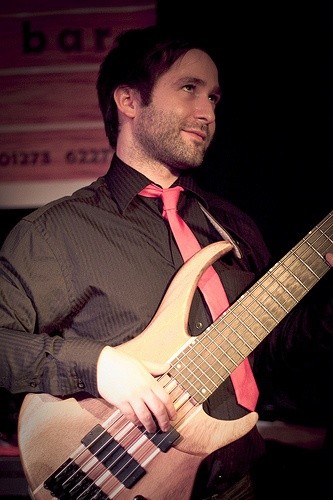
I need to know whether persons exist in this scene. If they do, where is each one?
[2,29,333,500]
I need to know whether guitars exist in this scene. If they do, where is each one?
[18,212,333,500]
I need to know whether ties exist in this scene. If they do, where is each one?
[140,185,259,412]
